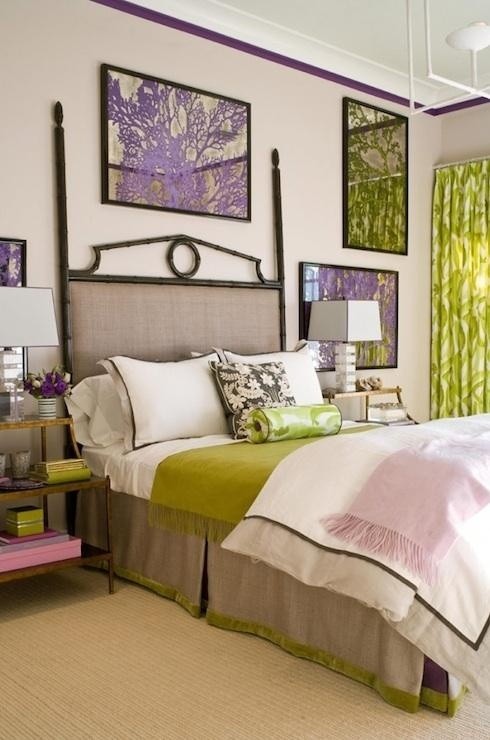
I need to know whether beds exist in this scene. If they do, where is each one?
[47,102,490,717]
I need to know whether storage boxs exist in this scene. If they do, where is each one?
[6,505,43,537]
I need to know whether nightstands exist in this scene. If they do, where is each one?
[322,387,419,426]
[1,415,113,593]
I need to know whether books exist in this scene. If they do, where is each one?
[29,458,91,484]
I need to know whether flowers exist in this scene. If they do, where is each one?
[22,364,72,399]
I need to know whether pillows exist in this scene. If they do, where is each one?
[96,351,233,454]
[210,340,323,405]
[247,404,341,444]
[210,360,297,440]
[64,375,124,448]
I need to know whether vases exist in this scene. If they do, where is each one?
[39,398,57,419]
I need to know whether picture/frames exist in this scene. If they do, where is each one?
[343,96,409,255]
[100,63,251,221]
[298,261,397,372]
[0,237,27,288]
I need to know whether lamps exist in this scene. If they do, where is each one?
[309,299,382,392]
[1,287,60,422]
[444,21,490,88]
